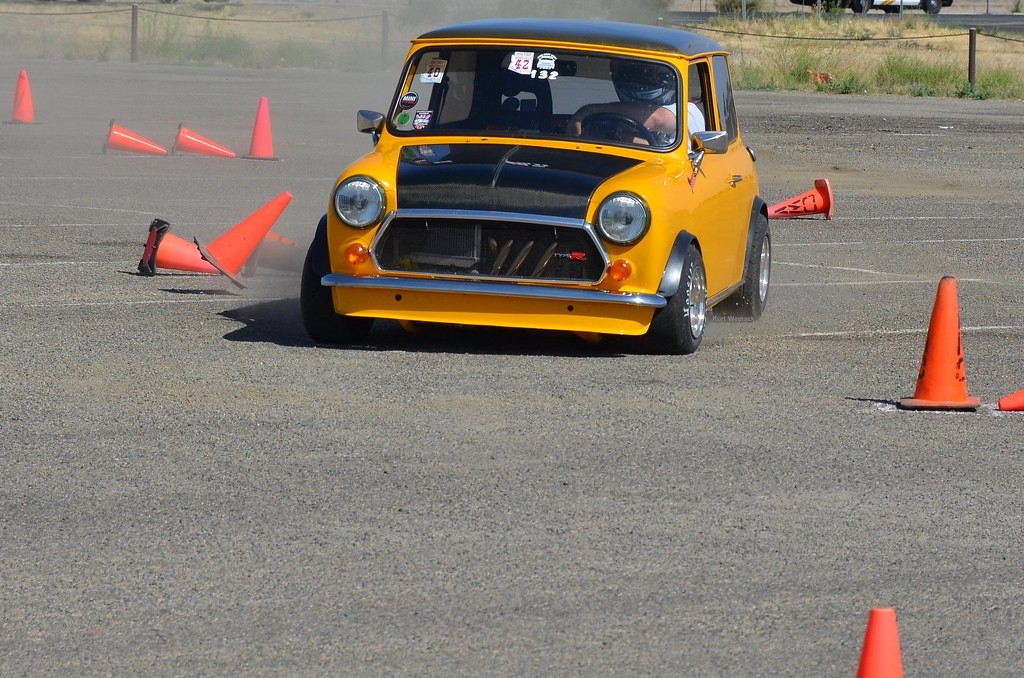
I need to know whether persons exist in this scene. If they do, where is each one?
[563,53,707,156]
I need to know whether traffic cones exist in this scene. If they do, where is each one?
[173,125,238,159]
[193,190,304,289]
[242,92,280,162]
[102,117,170,158]
[856,608,905,678]
[998,390,1024,412]
[137,217,223,279]
[7,68,43,127]
[767,178,835,221]
[895,275,984,412]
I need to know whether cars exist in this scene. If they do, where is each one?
[301,17,772,355]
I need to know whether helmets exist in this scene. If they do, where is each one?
[610,58,676,105]
